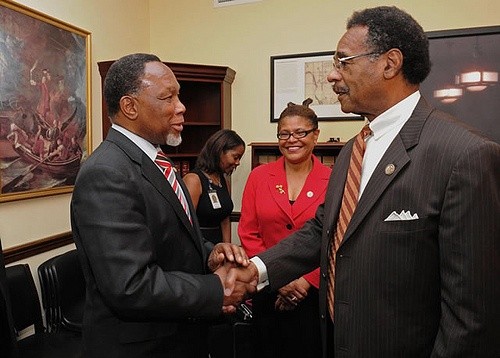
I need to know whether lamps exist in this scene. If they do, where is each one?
[431,37,498,107]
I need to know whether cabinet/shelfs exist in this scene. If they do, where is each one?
[97,60,237,180]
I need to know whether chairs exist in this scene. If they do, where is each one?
[6,250,88,358]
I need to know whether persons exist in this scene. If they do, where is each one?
[222,6,500,358]
[238,106,331,358]
[182,130,246,244]
[70,53,249,358]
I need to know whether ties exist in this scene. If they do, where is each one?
[156,152,193,228]
[326,124,374,325]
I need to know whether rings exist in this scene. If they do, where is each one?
[291,296,297,301]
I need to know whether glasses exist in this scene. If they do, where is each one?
[277,129,315,140]
[333,51,376,73]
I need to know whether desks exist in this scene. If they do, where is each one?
[247,138,347,178]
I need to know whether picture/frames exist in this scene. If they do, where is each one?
[419,24,500,148]
[0,0,93,201]
[270,49,364,123]
